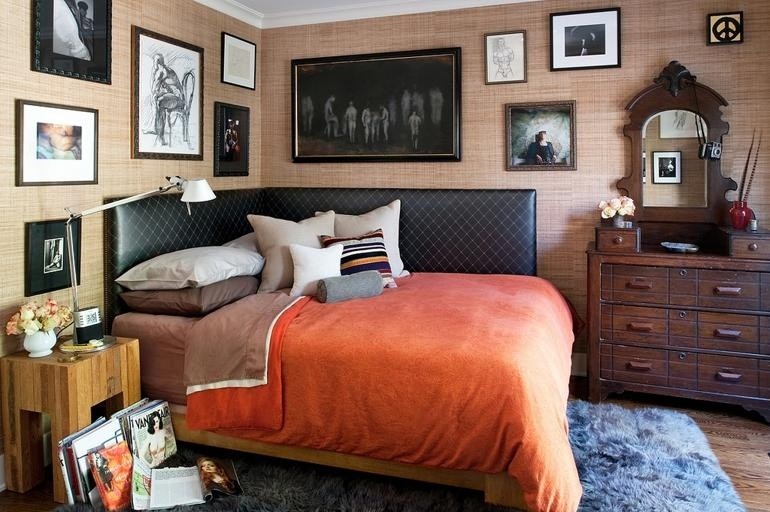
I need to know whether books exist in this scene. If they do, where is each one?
[57,397,245,512]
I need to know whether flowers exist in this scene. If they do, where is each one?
[7,301,71,333]
[598,196,635,218]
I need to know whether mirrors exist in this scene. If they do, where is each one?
[617,60,737,245]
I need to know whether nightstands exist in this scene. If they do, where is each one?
[0,335,141,504]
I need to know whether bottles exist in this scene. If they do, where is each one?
[751,219,757,231]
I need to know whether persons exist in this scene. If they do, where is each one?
[302,87,444,149]
[526,131,557,165]
[493,38,514,79]
[141,54,186,149]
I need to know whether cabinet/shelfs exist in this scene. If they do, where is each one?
[585,226,770,424]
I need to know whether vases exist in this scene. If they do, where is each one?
[614,212,626,228]
[24,332,57,358]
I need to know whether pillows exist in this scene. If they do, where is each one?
[115,199,410,313]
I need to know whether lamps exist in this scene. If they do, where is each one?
[60,175,217,351]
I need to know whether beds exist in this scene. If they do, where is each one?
[104,186,582,512]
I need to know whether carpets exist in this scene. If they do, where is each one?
[51,399,747,512]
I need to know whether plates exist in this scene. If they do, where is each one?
[658,240,701,254]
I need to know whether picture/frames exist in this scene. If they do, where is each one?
[15,96,101,186]
[707,11,744,44]
[27,1,116,89]
[210,100,253,178]
[292,46,462,163]
[659,111,707,140]
[505,100,578,170]
[24,217,81,296]
[550,8,621,70]
[128,21,207,165]
[653,151,682,184]
[219,29,256,91]
[484,29,527,84]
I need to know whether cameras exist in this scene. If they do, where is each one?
[698,143,707,159]
[706,141,722,160]
